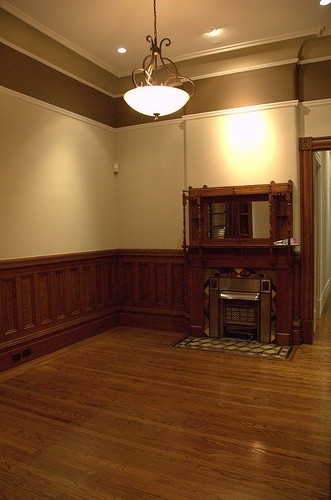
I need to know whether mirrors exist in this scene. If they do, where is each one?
[202,196,273,241]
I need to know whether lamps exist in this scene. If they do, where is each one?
[123,0,196,122]
